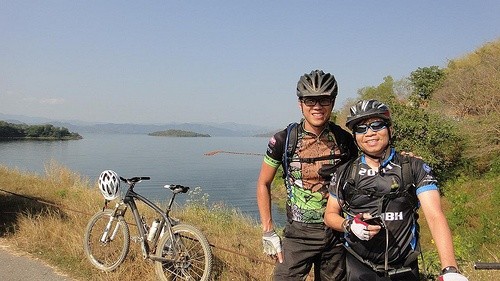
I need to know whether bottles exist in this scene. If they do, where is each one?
[147,218,160,243]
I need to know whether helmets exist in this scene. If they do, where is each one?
[98,170,121,200]
[297,70,338,97]
[346,99,391,130]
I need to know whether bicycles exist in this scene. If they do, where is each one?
[83,176,213,281]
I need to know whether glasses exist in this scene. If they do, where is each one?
[354,121,388,133]
[300,98,335,106]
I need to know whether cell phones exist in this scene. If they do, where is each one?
[363,217,385,231]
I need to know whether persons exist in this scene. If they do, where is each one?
[255,69,423,281]
[323,99,469,281]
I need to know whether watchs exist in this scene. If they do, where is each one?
[440,265,461,275]
